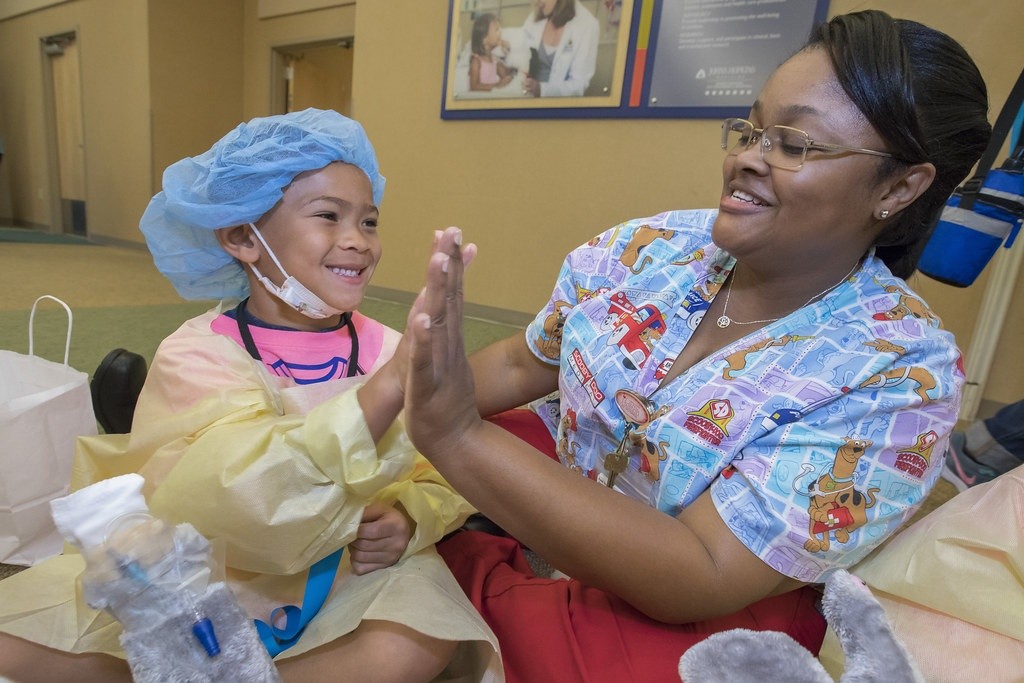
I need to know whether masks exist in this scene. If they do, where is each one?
[249,244,345,320]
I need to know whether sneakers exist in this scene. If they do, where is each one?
[940,431,998,493]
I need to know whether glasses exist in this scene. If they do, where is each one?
[720,118,937,168]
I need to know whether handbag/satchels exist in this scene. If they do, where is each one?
[0,295,99,569]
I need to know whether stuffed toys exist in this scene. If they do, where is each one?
[677,569,925,682]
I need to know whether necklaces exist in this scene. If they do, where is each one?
[716,258,859,328]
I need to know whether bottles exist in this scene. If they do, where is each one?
[977,146,1024,212]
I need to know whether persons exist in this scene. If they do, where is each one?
[467,13,512,91]
[0,9,994,683]
[507,0,601,97]
